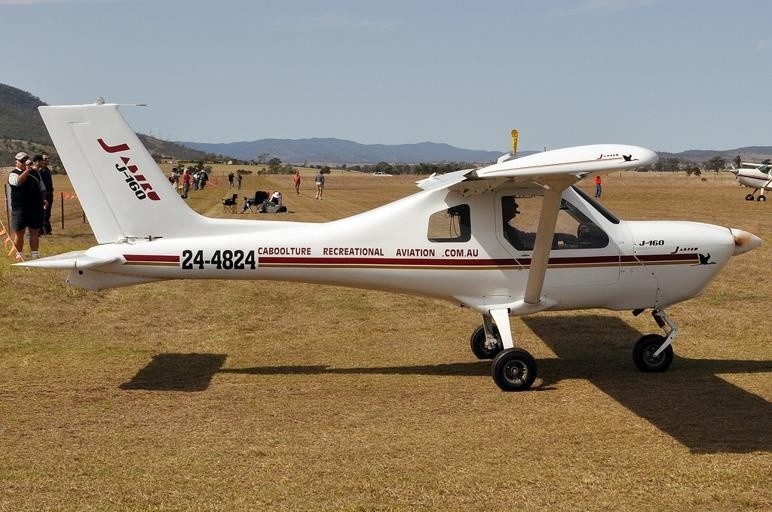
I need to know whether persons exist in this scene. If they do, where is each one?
[314,171,325,201]
[194,169,198,190]
[182,168,193,194]
[293,172,300,194]
[228,171,233,189]
[232,173,236,185]
[171,167,180,192]
[198,177,204,189]
[38,151,54,235]
[594,175,601,198]
[24,159,33,171]
[8,151,48,261]
[24,155,46,236]
[201,170,208,188]
[236,171,242,189]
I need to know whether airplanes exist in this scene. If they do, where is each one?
[12,103,763,392]
[729,163,772,202]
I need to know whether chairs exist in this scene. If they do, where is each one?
[222,193,238,214]
[243,190,266,214]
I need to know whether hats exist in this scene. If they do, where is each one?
[273,192,279,198]
[15,152,43,165]
[172,168,177,172]
